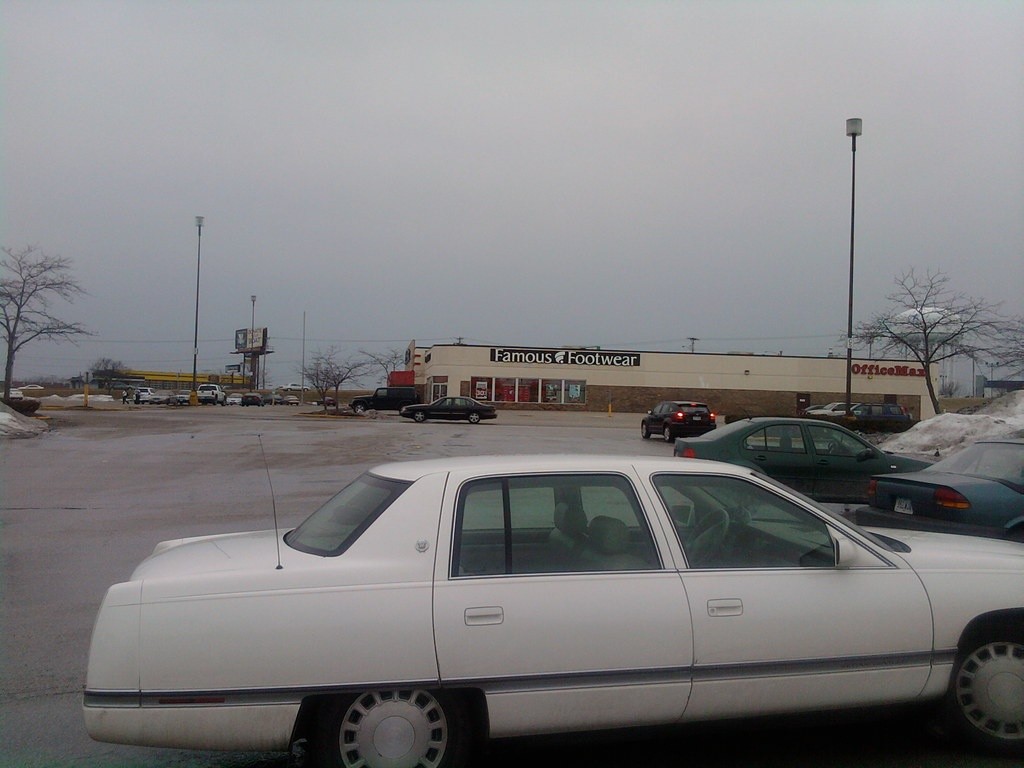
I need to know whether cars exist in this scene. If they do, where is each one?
[673,407,935,504]
[837,403,913,424]
[0,383,338,407]
[854,437,1023,533]
[805,401,862,416]
[640,400,717,442]
[399,396,497,425]
[81,434,1023,768]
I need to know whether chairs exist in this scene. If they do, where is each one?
[542,503,591,574]
[779,435,792,452]
[573,515,639,571]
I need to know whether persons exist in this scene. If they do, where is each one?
[135,388,141,401]
[122,389,129,404]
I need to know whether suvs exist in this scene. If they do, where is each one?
[347,386,418,414]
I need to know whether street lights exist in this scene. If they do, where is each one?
[250,294,257,392]
[188,214,203,406]
[845,116,863,415]
